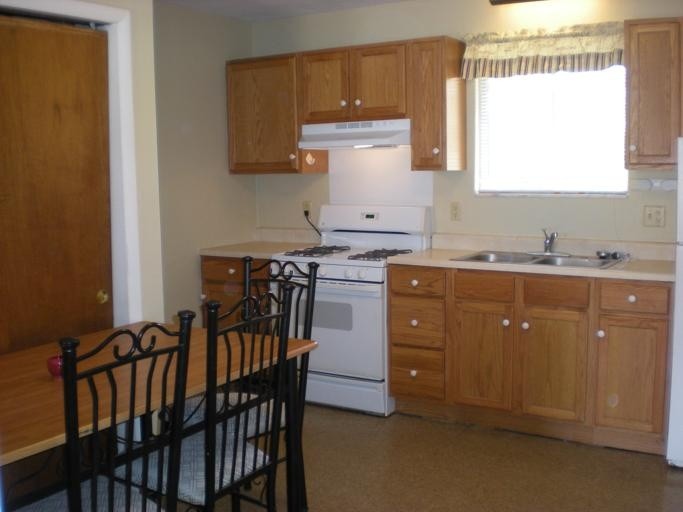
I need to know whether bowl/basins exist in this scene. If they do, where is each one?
[47,355,63,378]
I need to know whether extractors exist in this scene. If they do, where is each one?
[298,118,411,149]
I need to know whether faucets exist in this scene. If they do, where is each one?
[542,228,558,252]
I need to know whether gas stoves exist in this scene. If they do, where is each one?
[270,244,413,282]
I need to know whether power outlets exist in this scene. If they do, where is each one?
[300,198,314,220]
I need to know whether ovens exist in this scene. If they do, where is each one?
[270,276,396,417]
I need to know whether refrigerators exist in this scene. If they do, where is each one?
[664,136,682,470]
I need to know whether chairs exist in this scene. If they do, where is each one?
[182,255,321,512]
[101,280,297,512]
[2,304,196,512]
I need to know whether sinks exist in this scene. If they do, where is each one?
[532,255,622,270]
[449,250,538,265]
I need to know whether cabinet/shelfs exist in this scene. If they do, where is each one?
[224,53,329,175]
[448,268,591,428]
[410,35,467,173]
[384,261,446,405]
[199,255,270,336]
[624,14,679,167]
[592,275,671,435]
[301,40,409,124]
